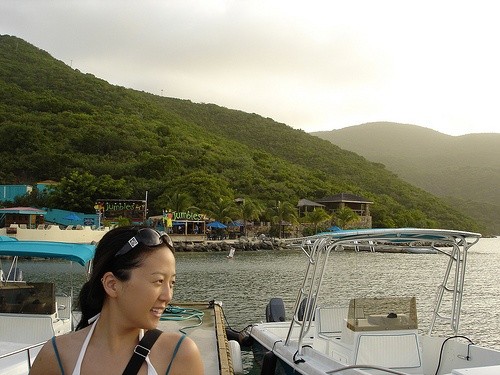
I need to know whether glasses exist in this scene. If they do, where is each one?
[115,229,173,258]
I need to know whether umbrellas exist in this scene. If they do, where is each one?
[206,221,228,241]
[225,218,253,238]
[325,225,342,232]
[274,219,293,240]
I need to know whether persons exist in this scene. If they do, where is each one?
[163,220,230,240]
[28,224,206,375]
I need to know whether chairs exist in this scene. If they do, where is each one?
[329,296,423,369]
[24,294,72,335]
[0,280,65,345]
[5,223,120,234]
[313,306,365,356]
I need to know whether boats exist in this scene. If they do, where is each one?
[1,235,242,375]
[245,225,499,375]
